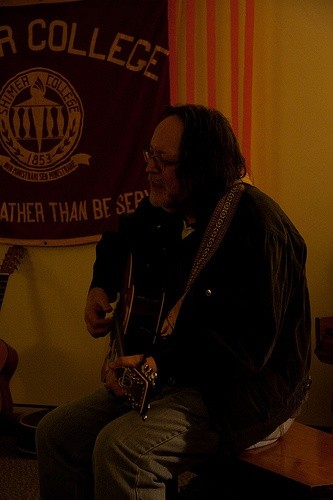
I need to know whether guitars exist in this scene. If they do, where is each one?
[1,243,28,443]
[101,258,156,417]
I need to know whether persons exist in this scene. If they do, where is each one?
[34,103,312,500]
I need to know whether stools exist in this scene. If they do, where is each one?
[165,420,333,499]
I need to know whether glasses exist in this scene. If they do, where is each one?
[144,145,178,172]
